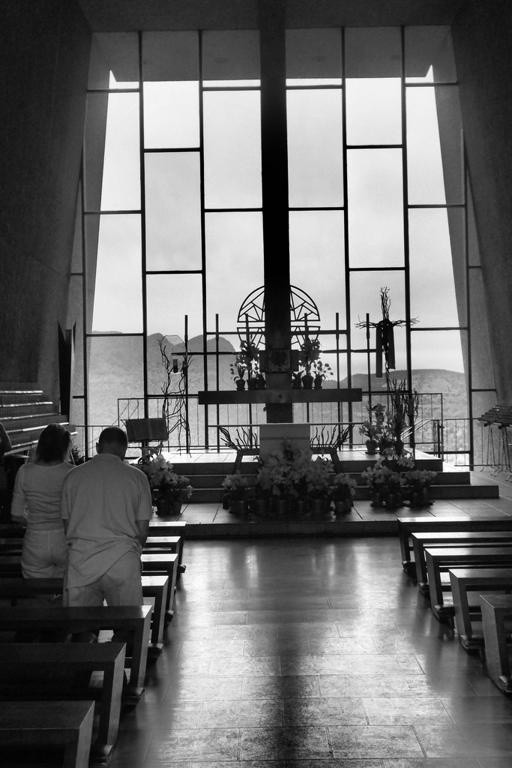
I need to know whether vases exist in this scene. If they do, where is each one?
[334,496,354,517]
[310,500,330,521]
[297,500,306,512]
[384,490,405,510]
[292,378,302,390]
[302,373,313,389]
[155,497,183,516]
[247,377,255,390]
[235,378,245,391]
[255,374,266,388]
[377,435,395,454]
[313,375,323,389]
[367,490,383,507]
[276,498,288,514]
[408,486,432,507]
[236,500,248,522]
[395,434,404,455]
[365,437,379,455]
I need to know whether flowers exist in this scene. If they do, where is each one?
[332,473,358,497]
[407,468,439,487]
[142,456,192,502]
[360,456,384,494]
[385,362,421,437]
[229,363,248,379]
[236,340,261,377]
[300,338,327,373]
[293,451,309,500]
[262,454,293,499]
[385,460,406,490]
[304,450,328,499]
[312,360,334,376]
[223,474,254,501]
[380,409,398,440]
[288,361,311,379]
[358,421,379,440]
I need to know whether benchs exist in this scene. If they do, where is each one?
[411,531,512,608]
[0,597,156,713]
[0,641,127,768]
[0,535,181,593]
[397,516,512,576]
[0,553,179,621]
[448,568,512,654]
[0,700,95,768]
[0,520,187,575]
[479,594,512,696]
[424,547,512,624]
[0,576,169,661]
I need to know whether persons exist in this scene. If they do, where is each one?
[11,423,77,579]
[60,426,151,634]
